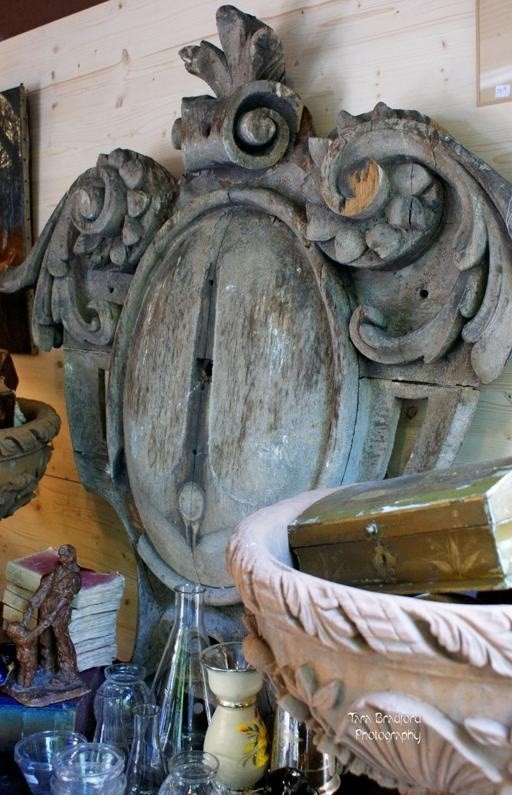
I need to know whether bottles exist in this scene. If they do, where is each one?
[94,583,272,795]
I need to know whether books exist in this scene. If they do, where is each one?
[2,546,126,673]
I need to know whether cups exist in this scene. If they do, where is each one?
[16,729,125,793]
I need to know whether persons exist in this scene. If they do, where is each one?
[6,605,49,689]
[29,544,80,687]
